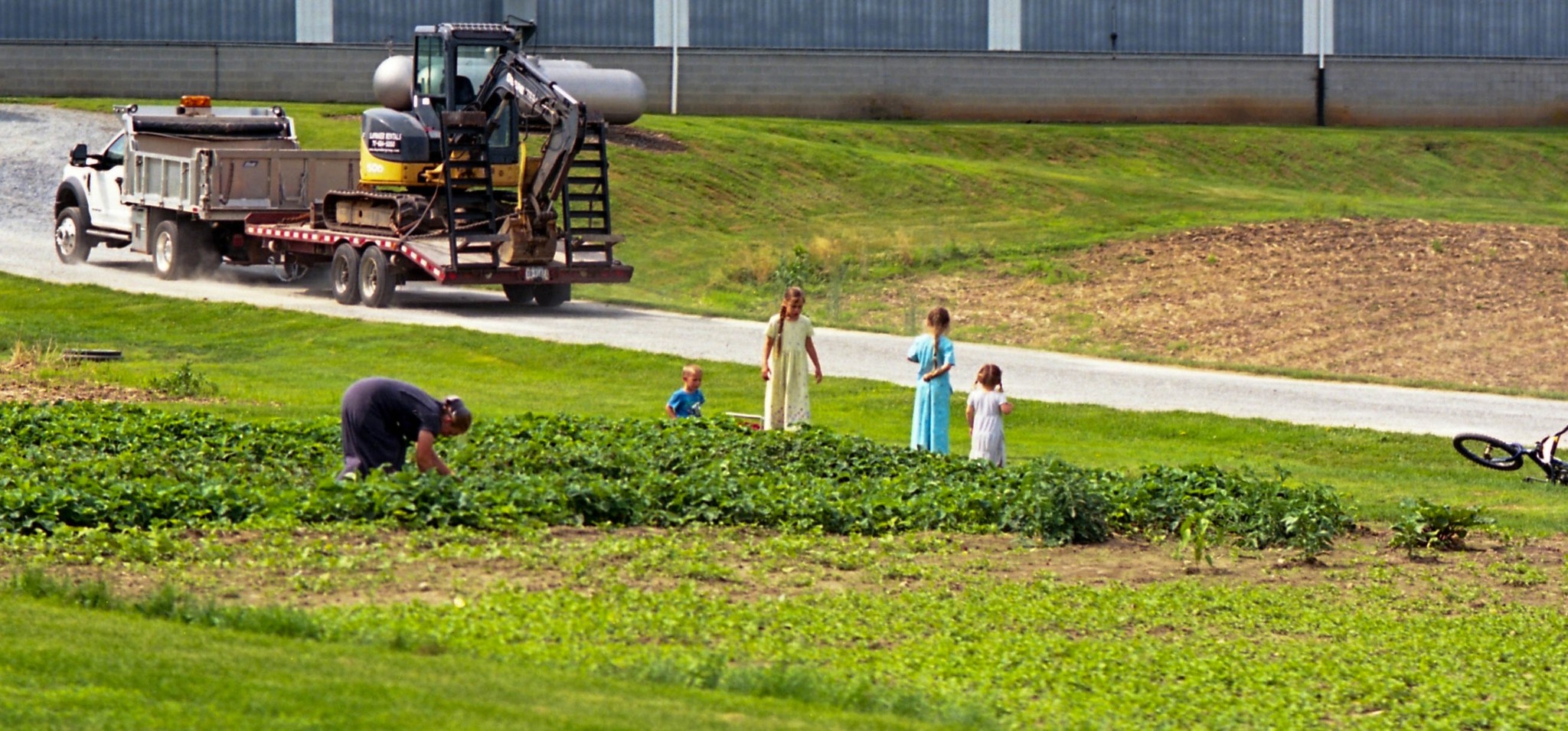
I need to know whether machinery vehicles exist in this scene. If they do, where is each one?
[323,13,588,265]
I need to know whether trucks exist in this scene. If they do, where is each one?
[54,95,373,281]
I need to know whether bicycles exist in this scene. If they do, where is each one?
[1453,425,1568,487]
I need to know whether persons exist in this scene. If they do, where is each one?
[762,287,823,431]
[907,307,956,455]
[334,376,472,485]
[967,364,1014,469]
[665,364,706,419]
